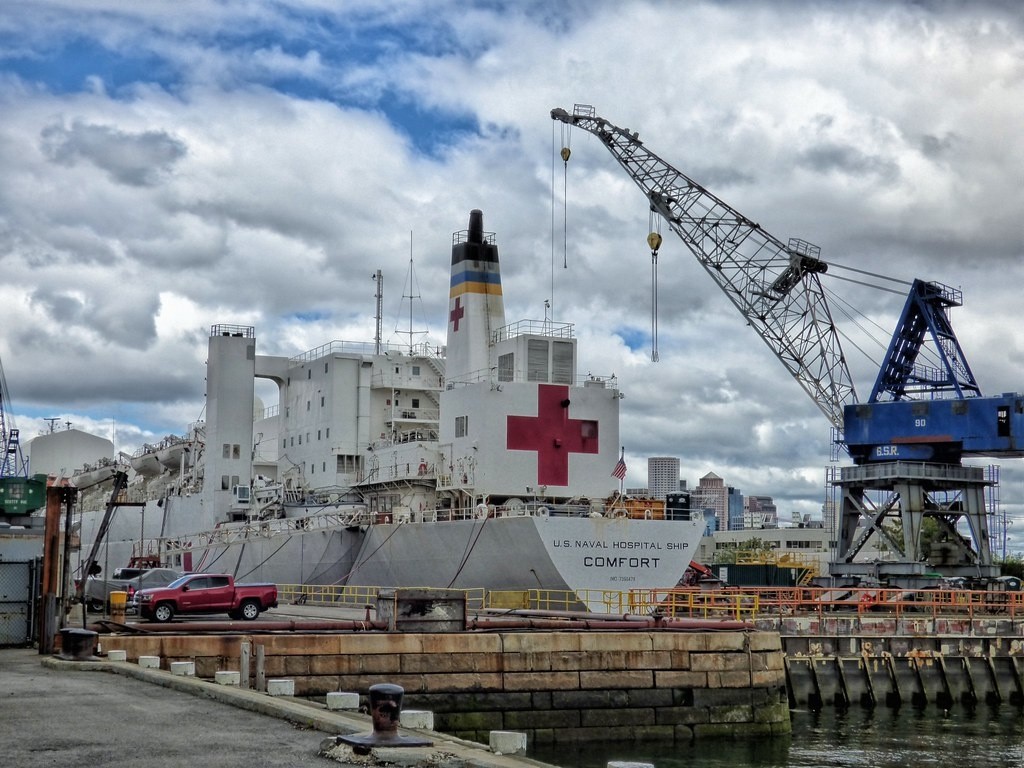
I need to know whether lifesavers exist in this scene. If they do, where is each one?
[613,507,629,518]
[418,463,428,475]
[476,503,489,519]
[861,594,873,608]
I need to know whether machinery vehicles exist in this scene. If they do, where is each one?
[551,107,1024,463]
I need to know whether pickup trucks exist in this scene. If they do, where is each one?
[134,573,280,623]
[76,563,185,614]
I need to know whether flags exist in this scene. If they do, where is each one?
[610,457,628,478]
